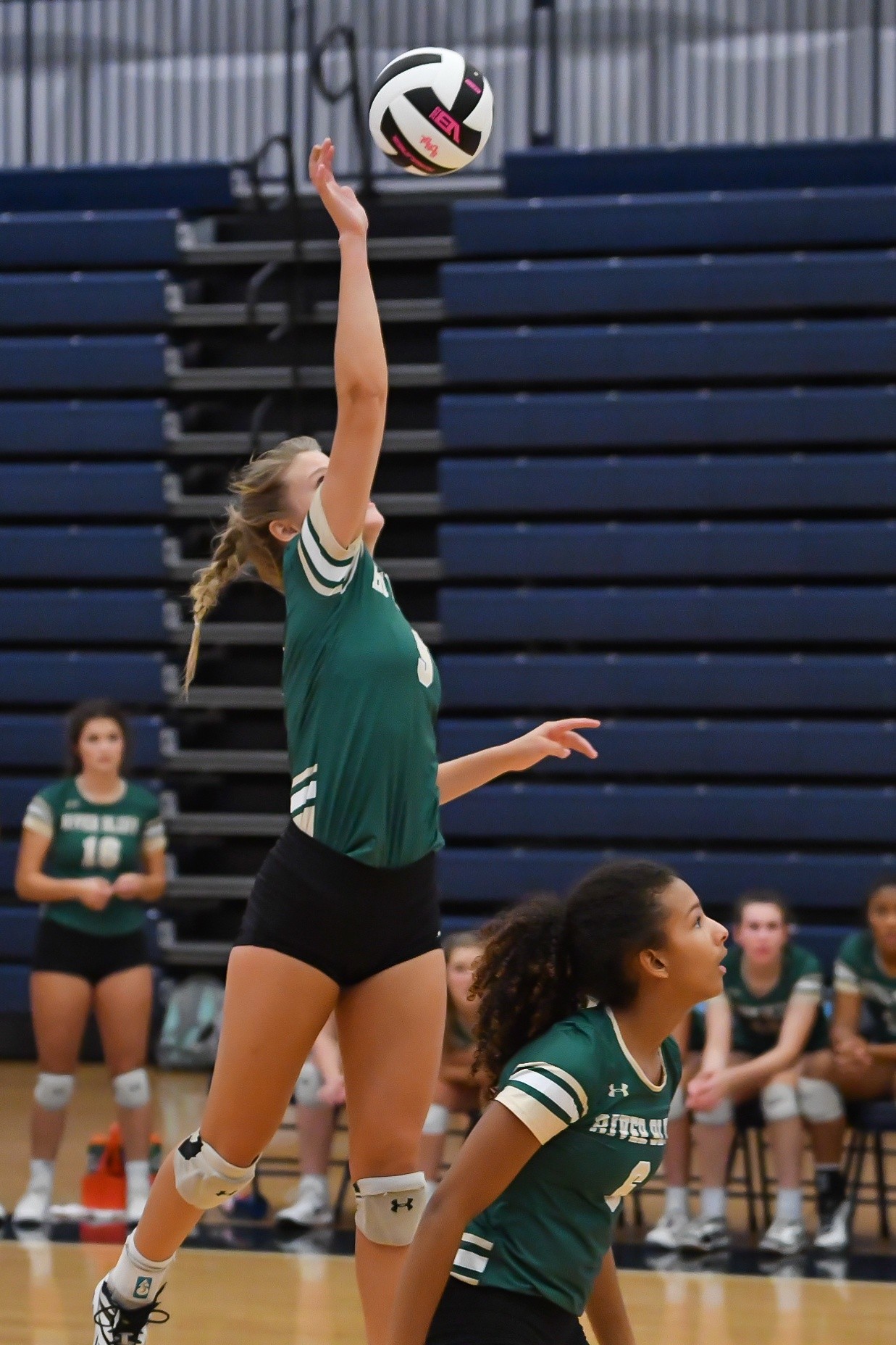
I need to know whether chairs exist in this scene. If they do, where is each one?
[187,989,896,1240]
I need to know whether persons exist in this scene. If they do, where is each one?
[390,858,729,1345]
[11,706,168,1229]
[93,136,603,1345]
[271,887,491,1231]
[675,890,834,1257]
[794,880,896,1254]
[644,1003,710,1250]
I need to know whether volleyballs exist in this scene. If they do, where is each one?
[368,46,495,178]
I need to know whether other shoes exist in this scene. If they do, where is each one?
[811,1169,852,1250]
[13,1231,49,1249]
[648,1252,682,1274]
[682,1253,727,1270]
[276,1229,332,1252]
[816,1257,848,1278]
[15,1195,46,1230]
[646,1214,687,1248]
[755,1219,806,1253]
[92,1274,169,1345]
[276,1193,331,1227]
[678,1215,729,1251]
[125,1199,147,1225]
[760,1256,804,1282]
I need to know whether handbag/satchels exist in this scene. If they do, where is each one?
[81,1123,158,1244]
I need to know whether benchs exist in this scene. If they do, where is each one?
[0,129,896,1048]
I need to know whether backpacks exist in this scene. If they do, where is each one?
[155,977,224,1068]
